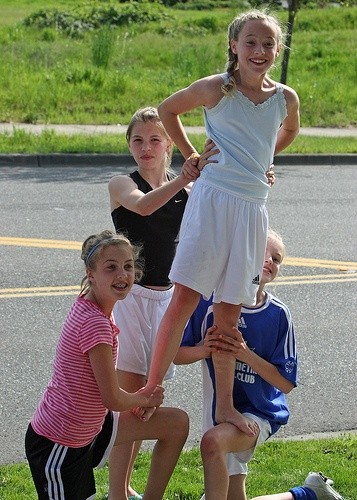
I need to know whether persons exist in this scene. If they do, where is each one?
[173,231,343,500]
[132,10,299,437]
[108,107,275,500]
[24,231,191,500]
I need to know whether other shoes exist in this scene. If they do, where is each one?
[303,470,343,500]
